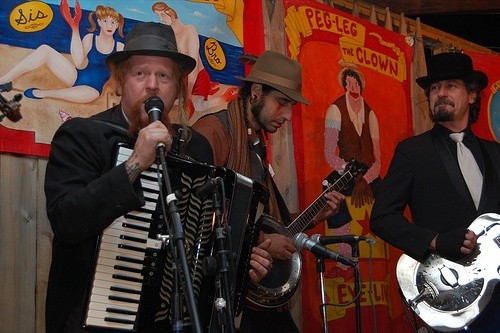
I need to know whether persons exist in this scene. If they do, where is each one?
[192,50,345,333]
[44,22,273,333]
[370,53,500,333]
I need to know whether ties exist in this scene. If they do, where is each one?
[450,132,483,211]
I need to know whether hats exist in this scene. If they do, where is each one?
[106,21,196,77]
[235,49,312,108]
[415,51,489,91]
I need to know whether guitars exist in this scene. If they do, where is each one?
[242,159,366,311]
[395,213,500,333]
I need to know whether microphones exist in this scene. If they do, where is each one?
[144,96,166,152]
[292,232,357,268]
[310,233,369,246]
